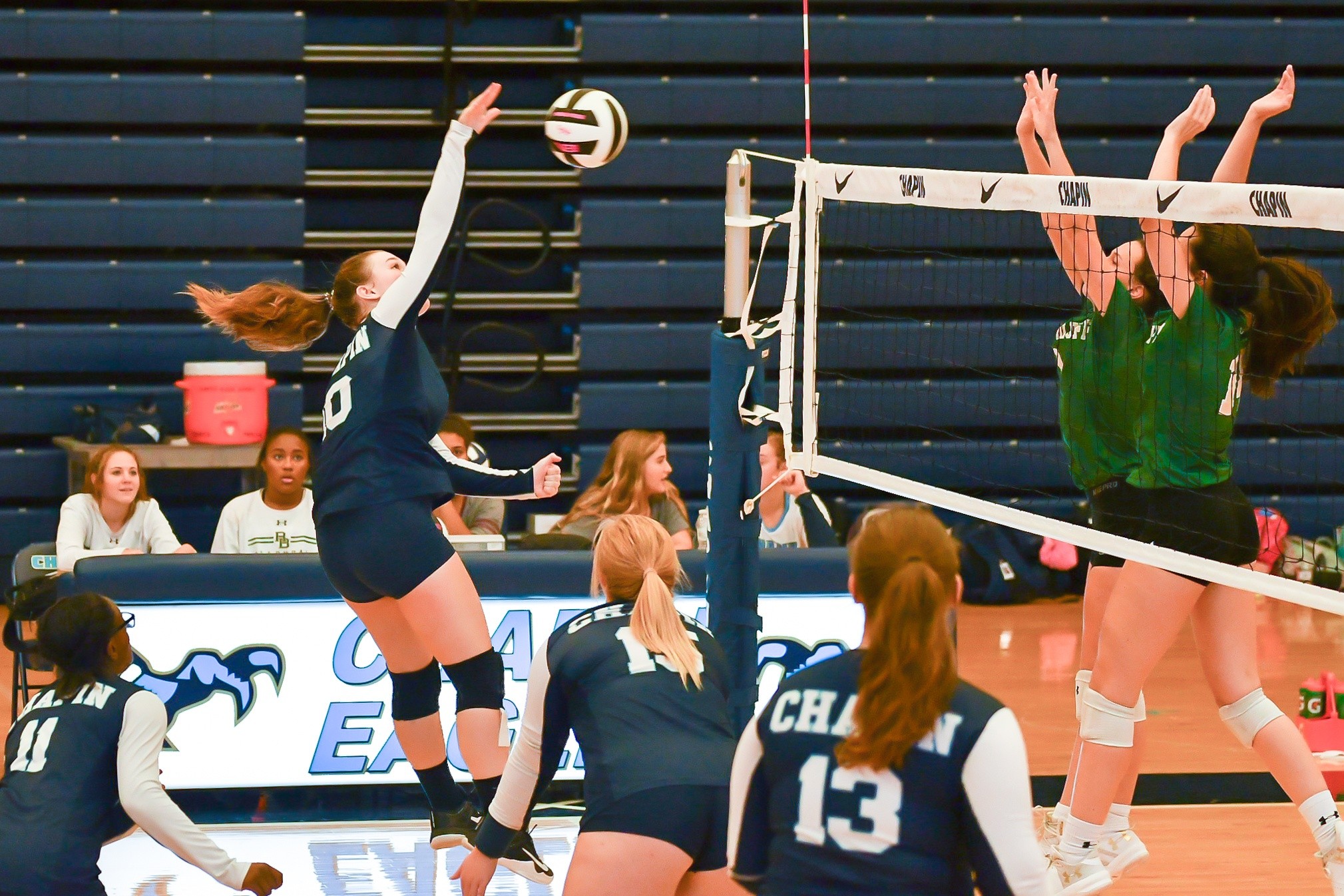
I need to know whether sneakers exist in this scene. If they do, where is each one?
[1033,804,1066,854]
[1097,825,1148,879]
[428,781,485,850]
[460,806,556,884]
[1042,843,1113,896]
[1312,820,1344,896]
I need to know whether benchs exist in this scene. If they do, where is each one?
[569,0,1344,618]
[0,9,308,555]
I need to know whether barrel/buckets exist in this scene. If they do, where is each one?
[176,360,275,444]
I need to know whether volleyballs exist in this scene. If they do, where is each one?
[544,89,628,168]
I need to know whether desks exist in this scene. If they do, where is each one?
[52,434,262,497]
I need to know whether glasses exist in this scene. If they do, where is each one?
[100,611,135,640]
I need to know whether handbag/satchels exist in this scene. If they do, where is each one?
[1275,535,1344,589]
[1249,506,1288,574]
[73,396,165,445]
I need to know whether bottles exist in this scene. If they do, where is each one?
[1298,682,1344,723]
[695,509,707,549]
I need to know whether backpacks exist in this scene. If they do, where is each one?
[960,522,1049,604]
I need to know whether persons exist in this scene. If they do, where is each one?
[1016,67,1344,895]
[431,412,504,535]
[56,446,197,576]
[170,82,563,884]
[0,594,284,896]
[549,429,691,550]
[728,499,1049,895]
[211,427,319,554]
[694,431,832,546]
[451,513,761,896]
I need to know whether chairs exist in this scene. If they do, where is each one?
[11,541,58,730]
